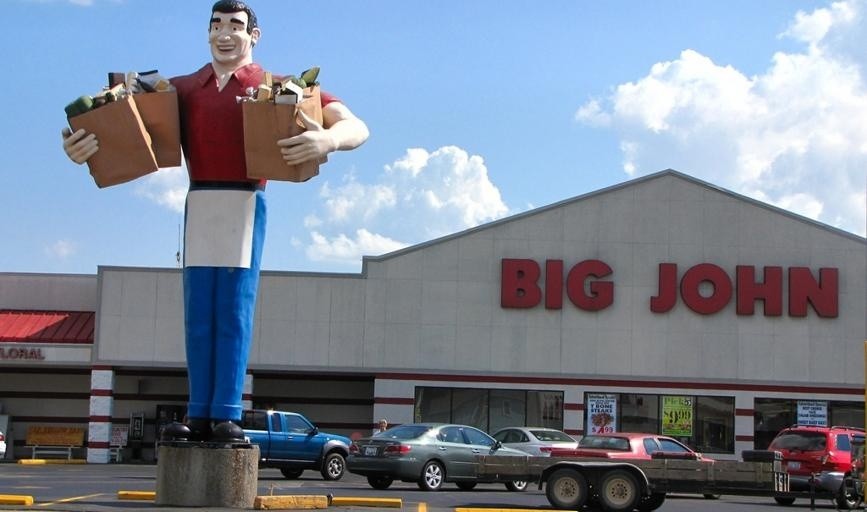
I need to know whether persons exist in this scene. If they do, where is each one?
[59,0,371,449]
[371,418,388,438]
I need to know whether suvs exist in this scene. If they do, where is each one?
[760,423,866,510]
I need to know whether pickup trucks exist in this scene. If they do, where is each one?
[153,408,355,482]
